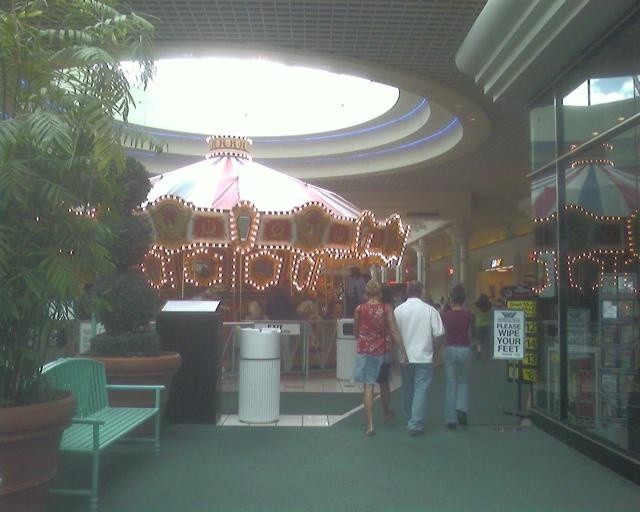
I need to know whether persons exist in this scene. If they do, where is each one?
[472,292,492,360]
[438,285,476,432]
[391,279,446,436]
[353,279,411,436]
[344,264,372,317]
[192,282,332,379]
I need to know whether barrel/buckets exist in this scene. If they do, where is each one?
[363,424,377,437]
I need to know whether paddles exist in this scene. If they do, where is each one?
[221,391,380,415]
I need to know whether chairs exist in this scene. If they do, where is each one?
[447,410,469,431]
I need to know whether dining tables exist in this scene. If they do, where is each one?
[0,0,167,512]
[73,155,180,437]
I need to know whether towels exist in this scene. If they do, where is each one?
[158,298,225,427]
[238,327,281,424]
[336,318,358,381]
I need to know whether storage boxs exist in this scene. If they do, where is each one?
[25,356,165,512]
